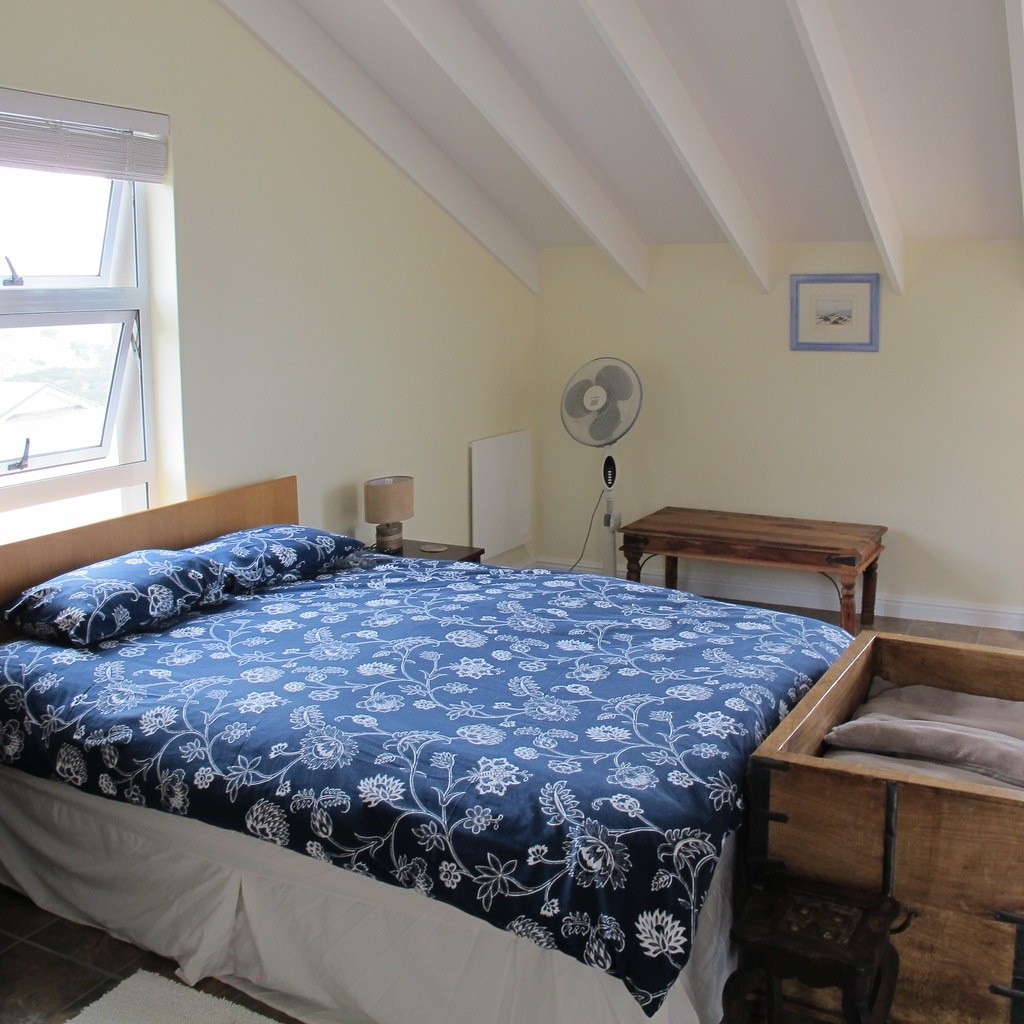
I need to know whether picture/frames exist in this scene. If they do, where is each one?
[788,272,881,352]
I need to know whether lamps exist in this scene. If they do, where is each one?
[364,475,414,551]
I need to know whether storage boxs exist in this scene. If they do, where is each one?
[747,630,1024,1024]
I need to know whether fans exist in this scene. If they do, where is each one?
[559,356,643,576]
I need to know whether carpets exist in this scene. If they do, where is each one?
[62,969,279,1024]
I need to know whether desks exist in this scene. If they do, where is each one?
[615,506,888,636]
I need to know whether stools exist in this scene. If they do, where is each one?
[719,878,901,1024]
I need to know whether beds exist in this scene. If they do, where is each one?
[0,474,855,1024]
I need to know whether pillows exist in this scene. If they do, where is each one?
[4,549,240,651]
[181,524,366,593]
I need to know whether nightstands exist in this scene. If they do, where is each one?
[375,538,485,564]
